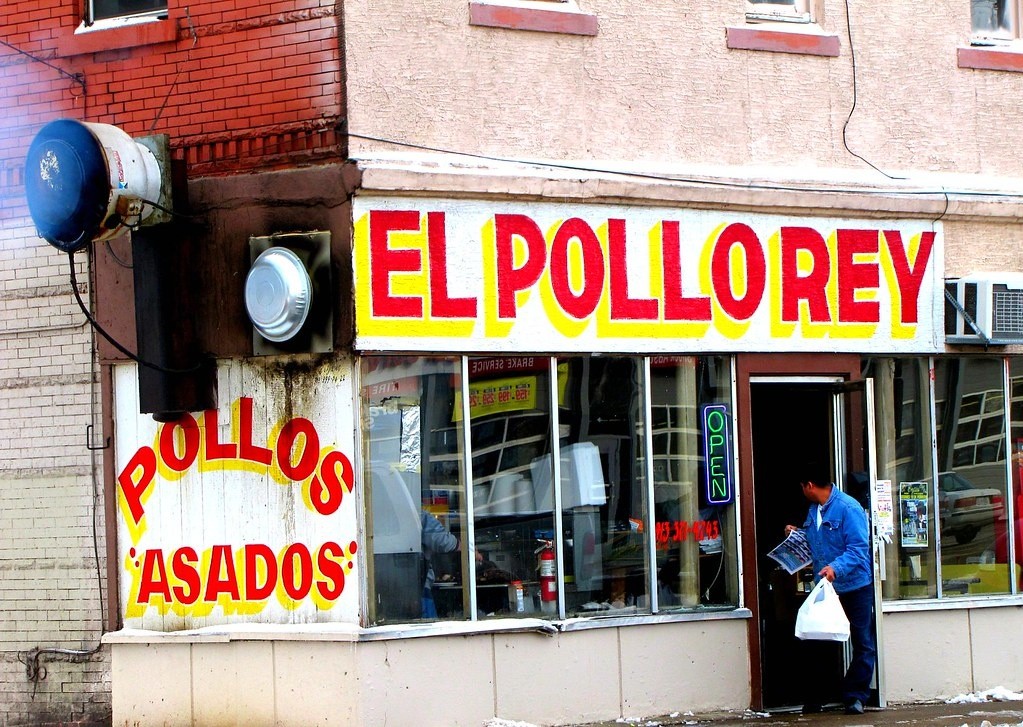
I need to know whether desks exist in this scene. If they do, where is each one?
[439,582,540,617]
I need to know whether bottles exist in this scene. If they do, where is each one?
[507,580,526,613]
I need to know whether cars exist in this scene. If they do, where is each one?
[895,469,1004,554]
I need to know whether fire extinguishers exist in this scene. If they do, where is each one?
[533,538,558,602]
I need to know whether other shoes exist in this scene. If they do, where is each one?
[847,700,866,714]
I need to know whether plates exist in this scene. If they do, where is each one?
[434,583,458,586]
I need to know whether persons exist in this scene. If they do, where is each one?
[784,467,877,714]
[421,509,482,619]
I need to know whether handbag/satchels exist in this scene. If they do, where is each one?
[795,576,850,642]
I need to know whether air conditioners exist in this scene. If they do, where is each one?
[955,273,1023,340]
